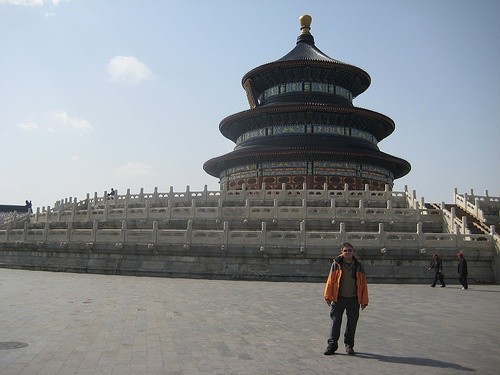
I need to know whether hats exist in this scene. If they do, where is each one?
[457,253,463,256]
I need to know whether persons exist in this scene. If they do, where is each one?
[428,253,446,287]
[25,200,33,212]
[456,253,468,289]
[108,188,116,199]
[323,242,368,355]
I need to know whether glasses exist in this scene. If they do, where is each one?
[342,249,353,252]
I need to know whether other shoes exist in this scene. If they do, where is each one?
[430,285,435,287]
[324,350,335,355]
[345,345,354,355]
[440,285,445,287]
[462,287,468,290]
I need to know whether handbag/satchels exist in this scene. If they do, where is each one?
[437,273,444,279]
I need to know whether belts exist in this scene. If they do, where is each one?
[339,296,355,299]
